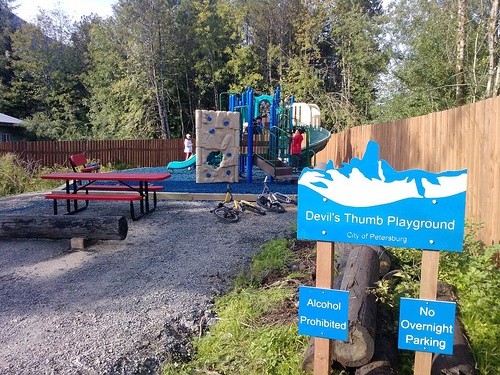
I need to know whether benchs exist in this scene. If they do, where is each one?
[43,192,143,221]
[78,183,163,208]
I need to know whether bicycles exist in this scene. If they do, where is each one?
[256,175,297,213]
[209,185,267,223]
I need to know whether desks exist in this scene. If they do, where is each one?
[40,173,171,216]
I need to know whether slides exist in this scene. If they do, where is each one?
[167,122,248,169]
[300,125,331,161]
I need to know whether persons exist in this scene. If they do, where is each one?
[255,117,263,134]
[184,134,194,170]
[244,118,258,134]
[290,129,304,174]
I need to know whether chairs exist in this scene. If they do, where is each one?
[70,154,98,182]
